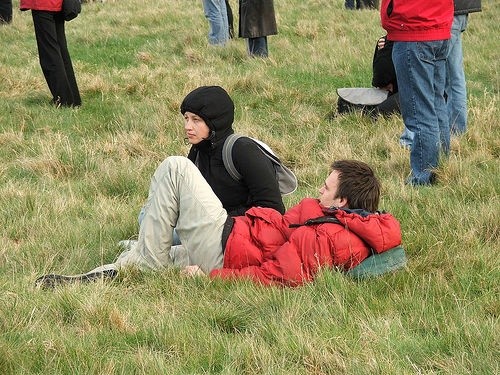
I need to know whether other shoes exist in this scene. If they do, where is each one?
[36,263,118,292]
[118,240,137,250]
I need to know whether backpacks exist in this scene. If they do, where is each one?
[288,216,409,278]
[222,134,300,195]
[62,0,81,21]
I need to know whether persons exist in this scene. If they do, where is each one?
[140,85,285,245]
[381,0,454,185]
[238,0,278,58]
[20,0,82,110]
[36,156,401,292]
[332,35,403,122]
[447,0,482,136]
[203,0,229,46]
